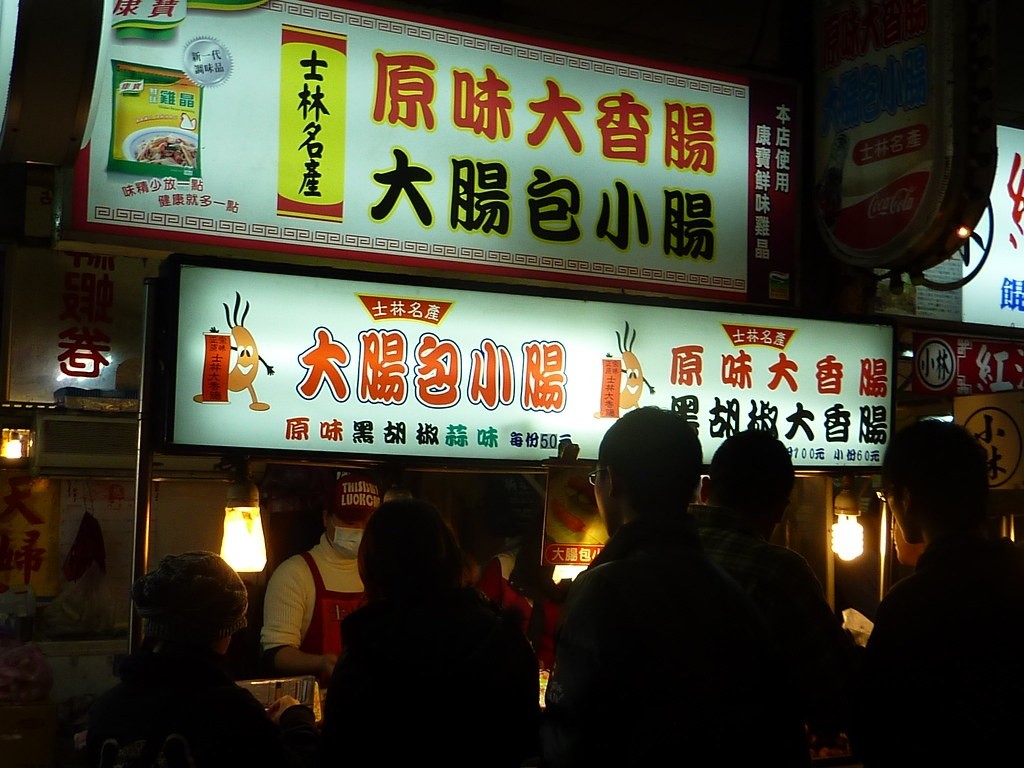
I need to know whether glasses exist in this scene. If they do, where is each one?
[587,467,617,486]
[874,486,907,502]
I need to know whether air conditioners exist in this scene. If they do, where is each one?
[29,414,238,483]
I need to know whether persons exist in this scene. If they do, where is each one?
[848,422,1024,768]
[322,500,541,768]
[541,403,867,768]
[87,551,321,768]
[261,474,381,689]
[478,506,572,675]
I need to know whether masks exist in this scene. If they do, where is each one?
[327,517,365,560]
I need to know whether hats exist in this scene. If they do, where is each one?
[133,551,248,641]
[333,476,382,524]
